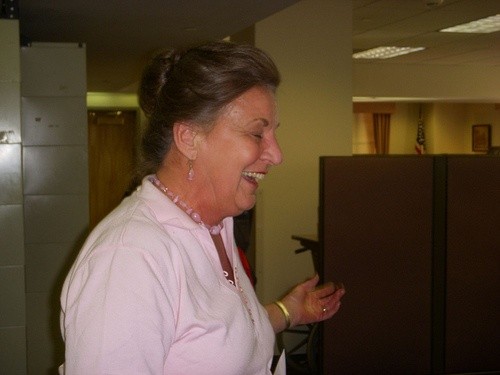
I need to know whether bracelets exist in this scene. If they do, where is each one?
[274,301,291,330]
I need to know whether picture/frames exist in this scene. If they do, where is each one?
[472,124,492,152]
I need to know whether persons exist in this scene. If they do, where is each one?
[58,40,346,375]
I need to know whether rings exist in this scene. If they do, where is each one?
[322,305,328,312]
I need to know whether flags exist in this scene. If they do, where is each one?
[414,115,428,154]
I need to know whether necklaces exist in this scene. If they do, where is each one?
[152,178,224,236]
